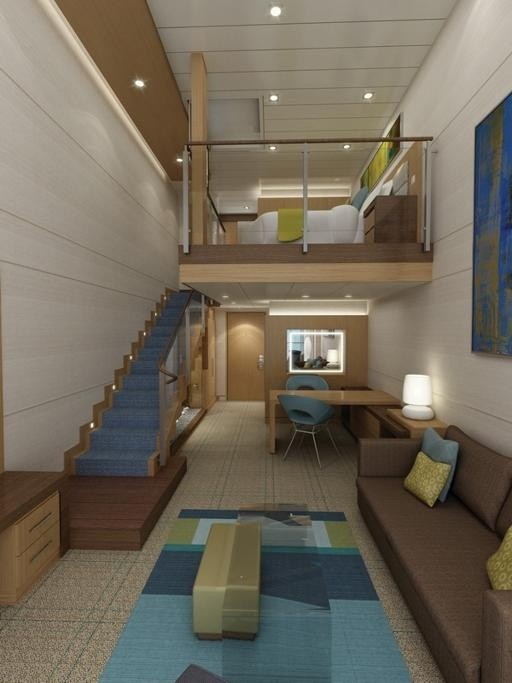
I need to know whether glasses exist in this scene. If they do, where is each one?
[402,374,434,420]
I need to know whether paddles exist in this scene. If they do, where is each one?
[98,509,412,683]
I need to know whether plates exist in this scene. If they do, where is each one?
[341,387,448,439]
[0,471,68,605]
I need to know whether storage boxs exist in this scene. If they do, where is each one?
[192,521,261,642]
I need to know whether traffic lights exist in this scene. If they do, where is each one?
[404,452,452,508]
[421,428,459,503]
[351,187,368,209]
[487,526,512,590]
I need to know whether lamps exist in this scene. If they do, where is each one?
[286,375,330,443]
[277,395,343,470]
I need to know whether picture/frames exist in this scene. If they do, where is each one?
[269,390,401,454]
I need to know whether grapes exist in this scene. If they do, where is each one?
[364,195,417,243]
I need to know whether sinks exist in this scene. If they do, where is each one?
[356,425,512,682]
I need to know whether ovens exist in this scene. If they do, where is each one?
[237,180,392,244]
